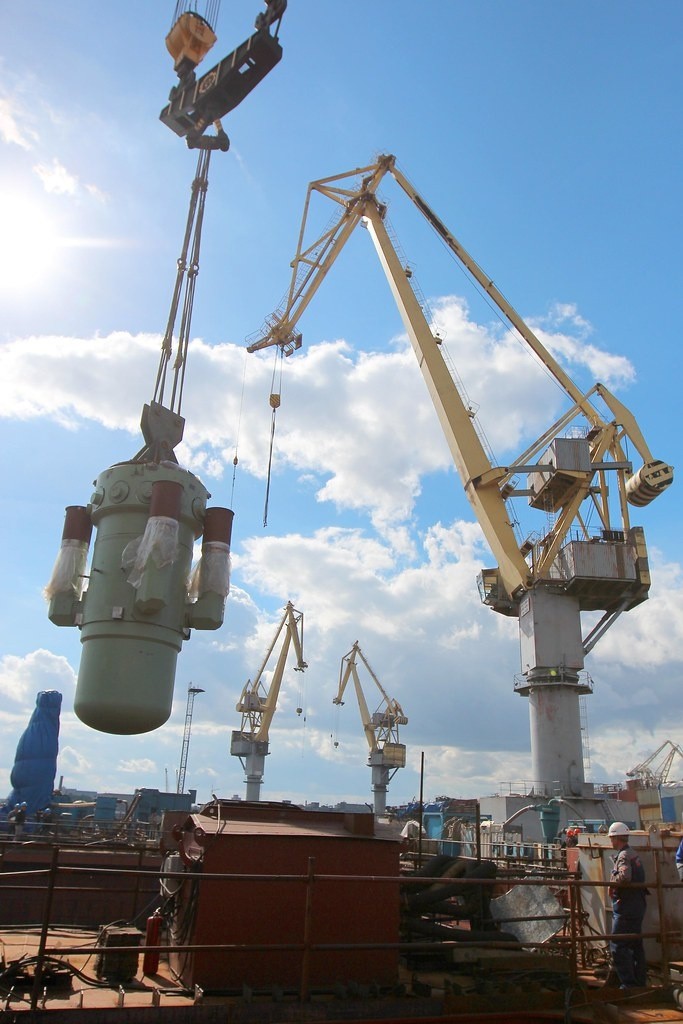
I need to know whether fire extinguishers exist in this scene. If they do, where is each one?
[141,907,164,979]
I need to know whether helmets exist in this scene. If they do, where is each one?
[21,802,27,806]
[15,803,20,809]
[45,809,51,813]
[606,822,631,837]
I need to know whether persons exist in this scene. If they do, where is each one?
[607,822,651,989]
[148,809,161,840]
[675,839,683,883]
[558,828,581,850]
[0,802,54,841]
